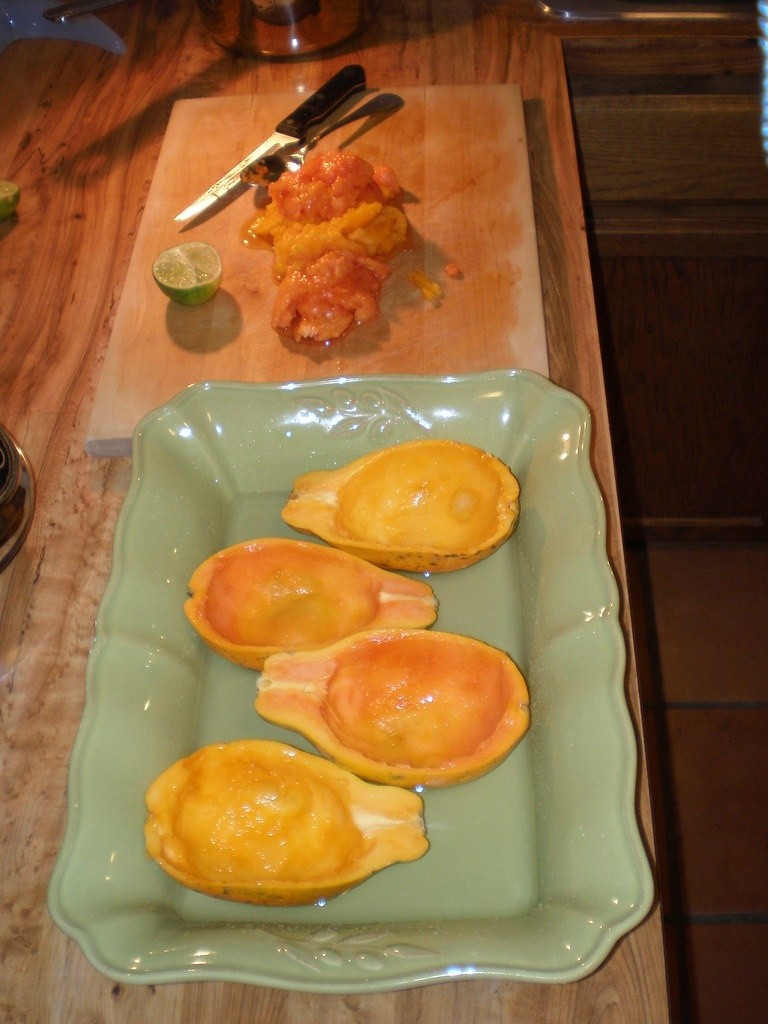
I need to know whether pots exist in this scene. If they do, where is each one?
[41,0,366,59]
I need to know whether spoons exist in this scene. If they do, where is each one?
[240,93,404,188]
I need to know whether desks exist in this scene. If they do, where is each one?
[0,0,768,1024]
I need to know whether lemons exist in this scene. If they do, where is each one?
[0,181,20,223]
[152,242,222,306]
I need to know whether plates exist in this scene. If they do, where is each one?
[45,367,656,995]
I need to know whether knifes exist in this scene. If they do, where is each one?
[174,64,368,222]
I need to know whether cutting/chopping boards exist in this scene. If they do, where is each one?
[84,82,550,460]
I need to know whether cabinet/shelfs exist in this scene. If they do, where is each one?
[562,32,767,538]
